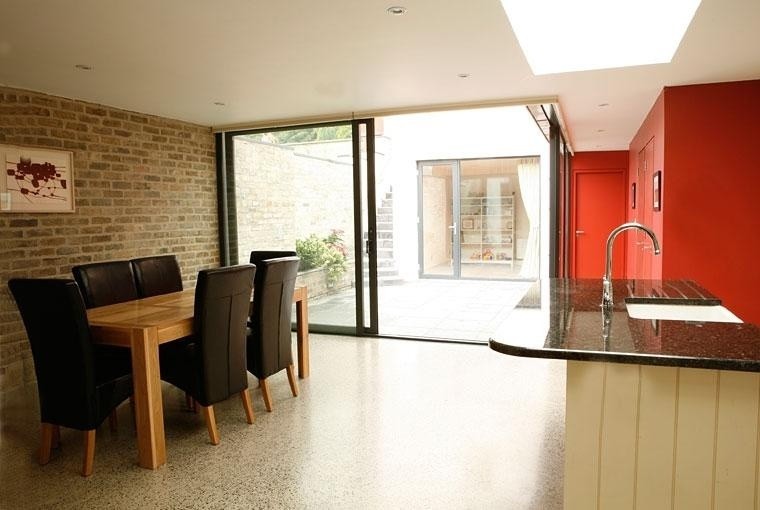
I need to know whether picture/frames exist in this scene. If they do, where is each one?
[632,183,636,209]
[652,170,661,212]
[0,141,76,213]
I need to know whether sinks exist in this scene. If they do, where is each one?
[626,303,745,323]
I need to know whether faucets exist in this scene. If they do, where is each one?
[602,222,660,306]
[602,306,611,352]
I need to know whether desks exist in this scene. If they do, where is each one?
[83,285,310,467]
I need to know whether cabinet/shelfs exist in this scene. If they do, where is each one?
[455,195,515,269]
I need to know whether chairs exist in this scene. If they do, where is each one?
[128,254,184,298]
[8,277,132,478]
[245,256,297,414]
[160,263,257,445]
[72,260,136,308]
[249,251,296,269]
